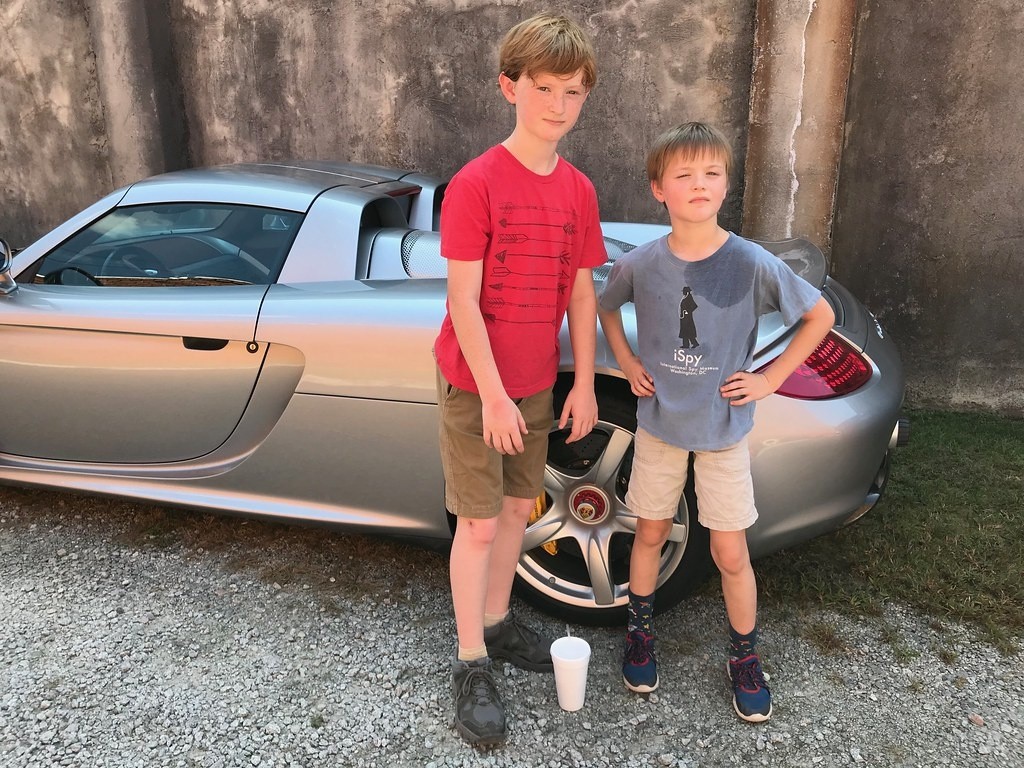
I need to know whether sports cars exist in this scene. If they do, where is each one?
[0,161,906,625]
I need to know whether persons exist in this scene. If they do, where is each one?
[597,123,836,723]
[432,12,609,745]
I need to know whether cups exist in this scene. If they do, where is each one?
[550,637,591,711]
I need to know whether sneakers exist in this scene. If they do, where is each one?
[483,611,555,671]
[726,657,772,722]
[622,629,659,693]
[450,645,508,745]
